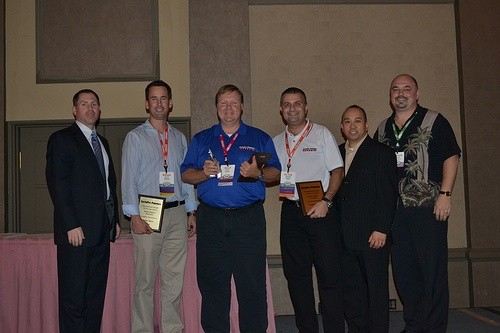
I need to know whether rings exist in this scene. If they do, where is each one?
[379,242,382,244]
[444,212,447,213]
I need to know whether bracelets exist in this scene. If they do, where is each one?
[187,212,196,216]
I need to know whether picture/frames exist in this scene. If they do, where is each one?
[35,0,160,84]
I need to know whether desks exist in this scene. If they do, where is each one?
[0,233,277,333]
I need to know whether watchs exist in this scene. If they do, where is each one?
[439,191,451,196]
[321,197,333,208]
[257,169,263,180]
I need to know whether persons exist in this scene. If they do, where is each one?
[121,81,197,333]
[180,85,282,333]
[330,105,397,333]
[46,89,121,333]
[273,87,344,333]
[374,74,461,333]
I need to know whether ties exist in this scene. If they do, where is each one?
[91,131,107,203]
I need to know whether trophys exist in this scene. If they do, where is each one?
[208,149,222,178]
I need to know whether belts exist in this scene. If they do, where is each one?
[164,200,185,209]
[201,201,263,212]
[283,199,300,208]
